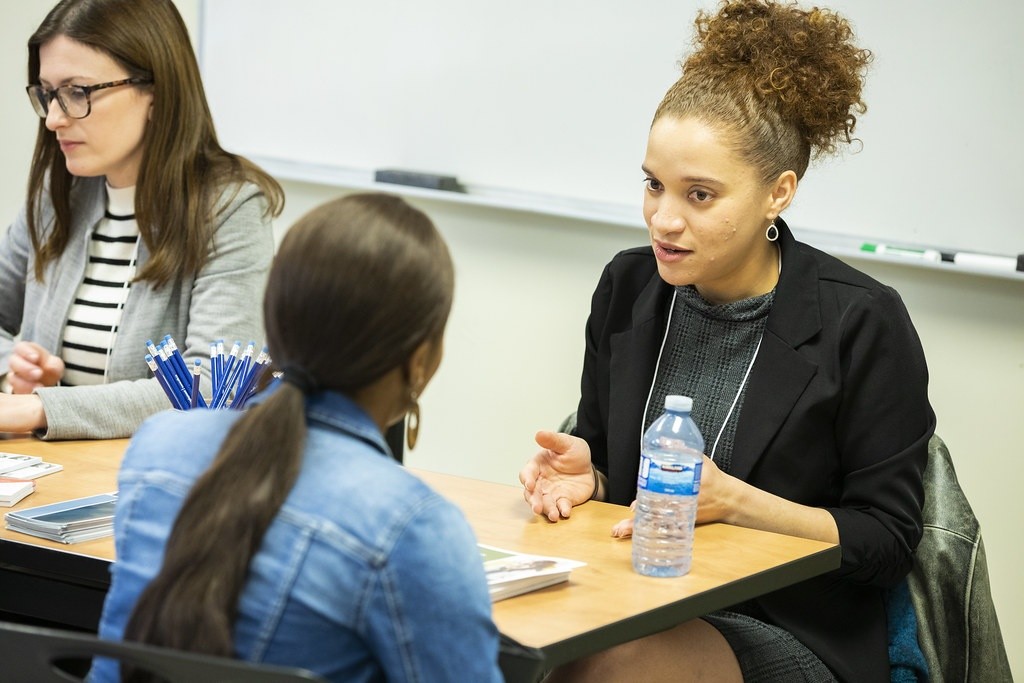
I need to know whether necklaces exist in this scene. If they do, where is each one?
[641,246,781,483]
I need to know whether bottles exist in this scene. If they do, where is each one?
[626,394,703,579]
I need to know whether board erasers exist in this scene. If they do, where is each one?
[373,165,462,199]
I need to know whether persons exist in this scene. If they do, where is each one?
[521,0,938,683]
[0,1,280,440]
[81,193,507,683]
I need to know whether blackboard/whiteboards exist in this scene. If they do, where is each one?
[182,1,1024,284]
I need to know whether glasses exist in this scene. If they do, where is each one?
[26,77,154,119]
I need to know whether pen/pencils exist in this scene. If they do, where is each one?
[143,332,272,412]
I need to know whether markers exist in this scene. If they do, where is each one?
[938,245,1024,280]
[858,241,942,270]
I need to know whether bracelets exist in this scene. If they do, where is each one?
[587,463,601,504]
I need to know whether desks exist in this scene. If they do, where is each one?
[0,435,843,683]
